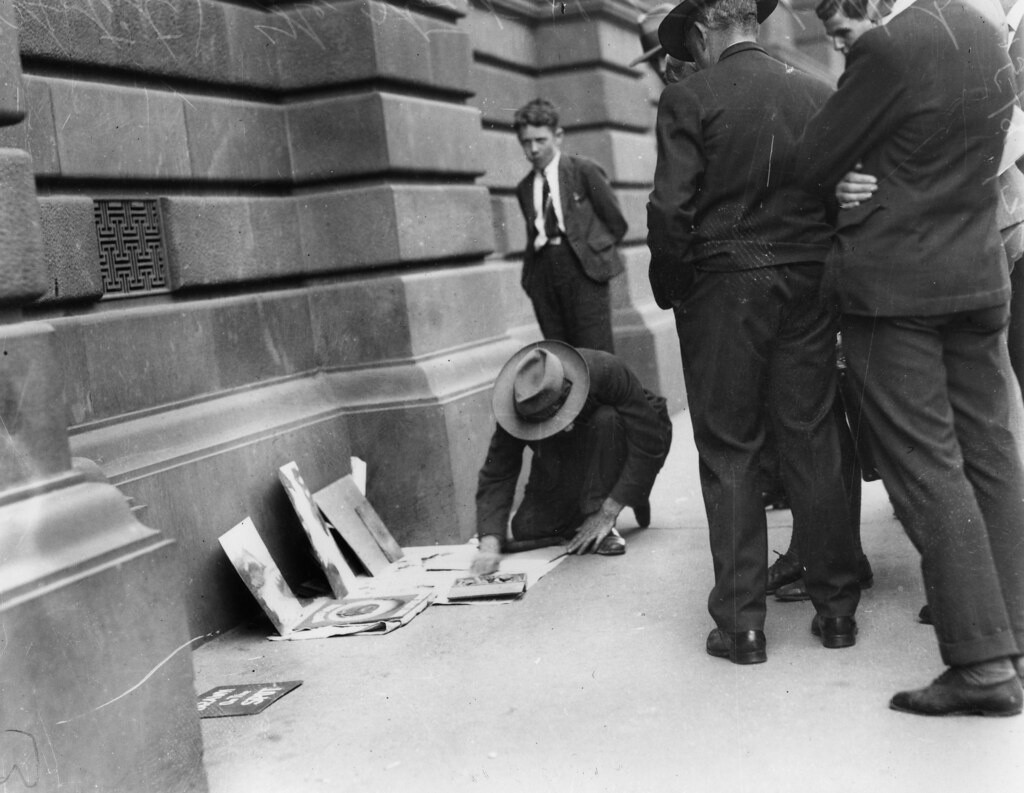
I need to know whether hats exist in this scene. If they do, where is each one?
[629,3,675,68]
[491,339,590,439]
[658,0,778,62]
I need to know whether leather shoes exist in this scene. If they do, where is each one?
[766,547,803,594]
[595,526,626,555]
[889,668,1024,717]
[776,553,874,600]
[706,626,767,664]
[811,613,858,648]
[632,490,651,527]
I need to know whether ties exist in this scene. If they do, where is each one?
[540,171,561,245]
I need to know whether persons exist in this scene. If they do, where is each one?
[466,338,673,578]
[629,1,1024,719]
[514,96,630,357]
